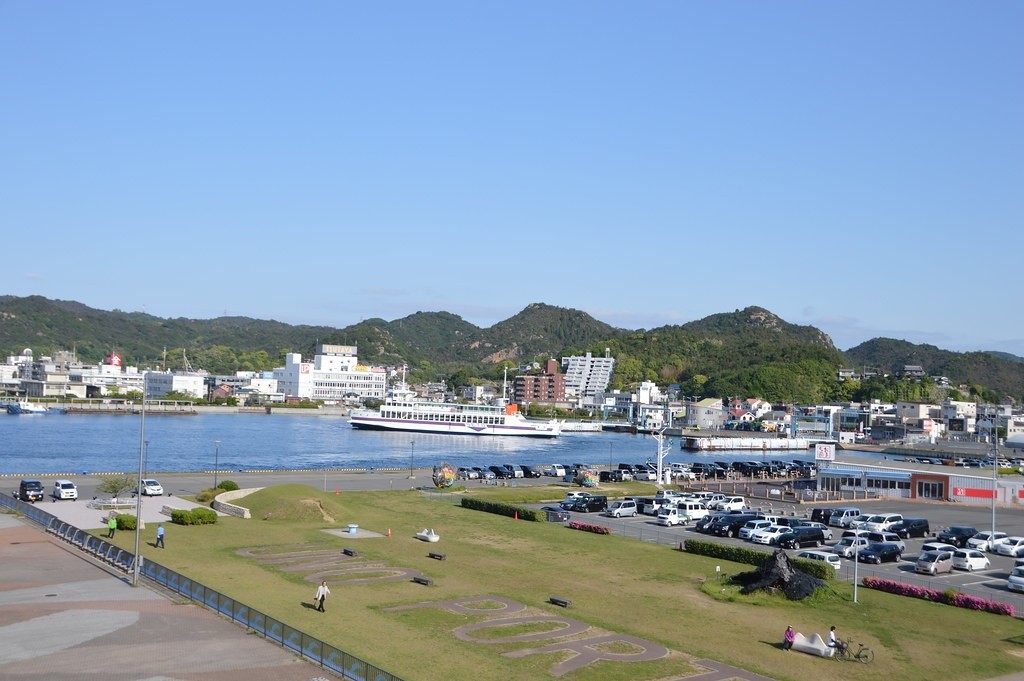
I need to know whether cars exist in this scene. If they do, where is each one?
[137,479,163,496]
[455,458,1024,594]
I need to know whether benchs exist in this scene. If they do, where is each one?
[429,551,446,560]
[343,548,356,557]
[413,575,433,586]
[790,632,834,658]
[550,597,572,608]
[416,528,440,542]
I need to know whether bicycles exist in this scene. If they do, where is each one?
[834,636,874,665]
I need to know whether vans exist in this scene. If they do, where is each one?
[19,480,44,502]
[53,480,78,500]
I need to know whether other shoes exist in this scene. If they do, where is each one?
[783,649,787,652]
[108,535,109,537]
[323,610,325,612]
[110,537,113,539]
[317,610,321,611]
[788,649,790,651]
[154,545,158,548]
[161,547,164,549]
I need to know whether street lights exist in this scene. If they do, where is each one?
[144,440,151,479]
[407,441,416,480]
[213,440,222,490]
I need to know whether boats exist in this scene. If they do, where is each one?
[347,385,560,438]
[7,401,48,415]
[548,418,603,432]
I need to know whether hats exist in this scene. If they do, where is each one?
[788,625,793,628]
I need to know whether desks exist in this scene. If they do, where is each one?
[347,524,358,534]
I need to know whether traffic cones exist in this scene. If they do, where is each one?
[387,528,392,537]
[514,510,518,520]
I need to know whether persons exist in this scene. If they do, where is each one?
[782,624,795,651]
[314,581,331,613]
[108,516,116,539]
[828,625,845,655]
[155,525,165,549]
